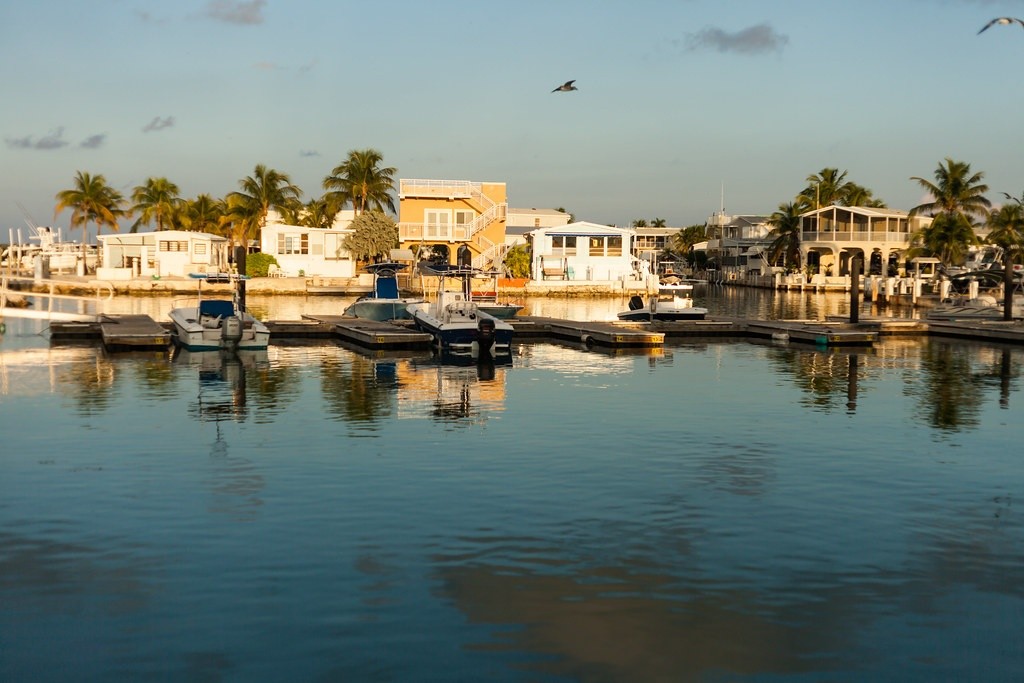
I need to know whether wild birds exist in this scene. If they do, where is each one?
[976,17,1024,34]
[552,79,579,93]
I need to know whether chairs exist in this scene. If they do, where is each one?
[268,264,288,278]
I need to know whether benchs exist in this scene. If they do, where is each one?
[542,268,569,280]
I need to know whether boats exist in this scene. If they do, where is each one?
[926,268,1024,321]
[166,271,272,352]
[405,262,516,364]
[467,271,525,318]
[342,261,430,321]
[863,275,915,300]
[658,260,685,284]
[617,284,709,321]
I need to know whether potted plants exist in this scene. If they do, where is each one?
[804,264,816,283]
[784,260,796,276]
[824,263,833,276]
